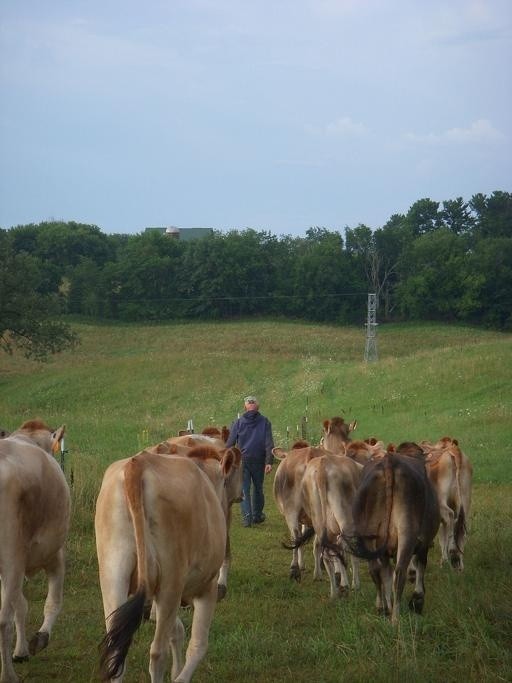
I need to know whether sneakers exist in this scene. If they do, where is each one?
[241,512,266,527]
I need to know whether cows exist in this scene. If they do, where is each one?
[271,416,473,629]
[0,419,72,682]
[94,424,241,683]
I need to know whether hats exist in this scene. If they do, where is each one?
[244,396,257,404]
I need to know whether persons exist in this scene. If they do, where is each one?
[221,396,276,529]
